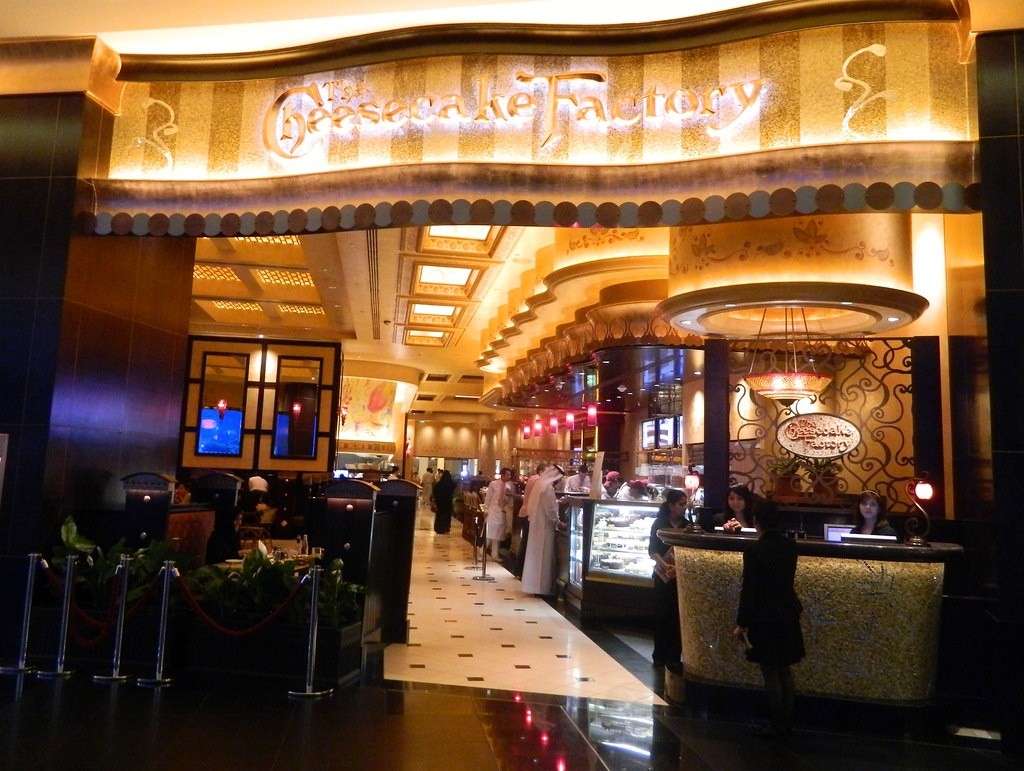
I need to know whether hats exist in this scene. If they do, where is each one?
[604,471,620,487]
[628,481,645,491]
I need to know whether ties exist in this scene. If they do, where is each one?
[498,483,505,506]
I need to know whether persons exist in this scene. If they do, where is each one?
[600,469,705,502]
[387,466,445,509]
[315,525,369,584]
[732,502,807,732]
[339,474,346,480]
[476,463,592,598]
[248,475,268,512]
[205,505,245,565]
[711,482,756,530]
[851,490,898,536]
[177,483,192,505]
[432,470,455,534]
[649,490,693,672]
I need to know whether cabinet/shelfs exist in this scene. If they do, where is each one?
[565,496,705,628]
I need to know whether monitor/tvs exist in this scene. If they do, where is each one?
[824,524,898,544]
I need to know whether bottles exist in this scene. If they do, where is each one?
[296,537,302,555]
[302,535,308,554]
[272,546,289,562]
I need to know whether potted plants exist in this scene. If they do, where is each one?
[769,459,800,495]
[805,458,844,494]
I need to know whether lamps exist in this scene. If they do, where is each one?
[534,392,542,437]
[523,398,532,439]
[549,385,559,433]
[742,305,834,400]
[565,376,575,431]
[586,366,598,426]
[902,470,937,547]
[683,463,705,533]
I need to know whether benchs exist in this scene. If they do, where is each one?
[164,509,216,575]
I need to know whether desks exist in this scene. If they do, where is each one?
[216,559,310,579]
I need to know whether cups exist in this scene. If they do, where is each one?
[313,548,324,554]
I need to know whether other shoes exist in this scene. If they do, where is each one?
[751,719,794,739]
[492,556,503,562]
[477,555,483,562]
